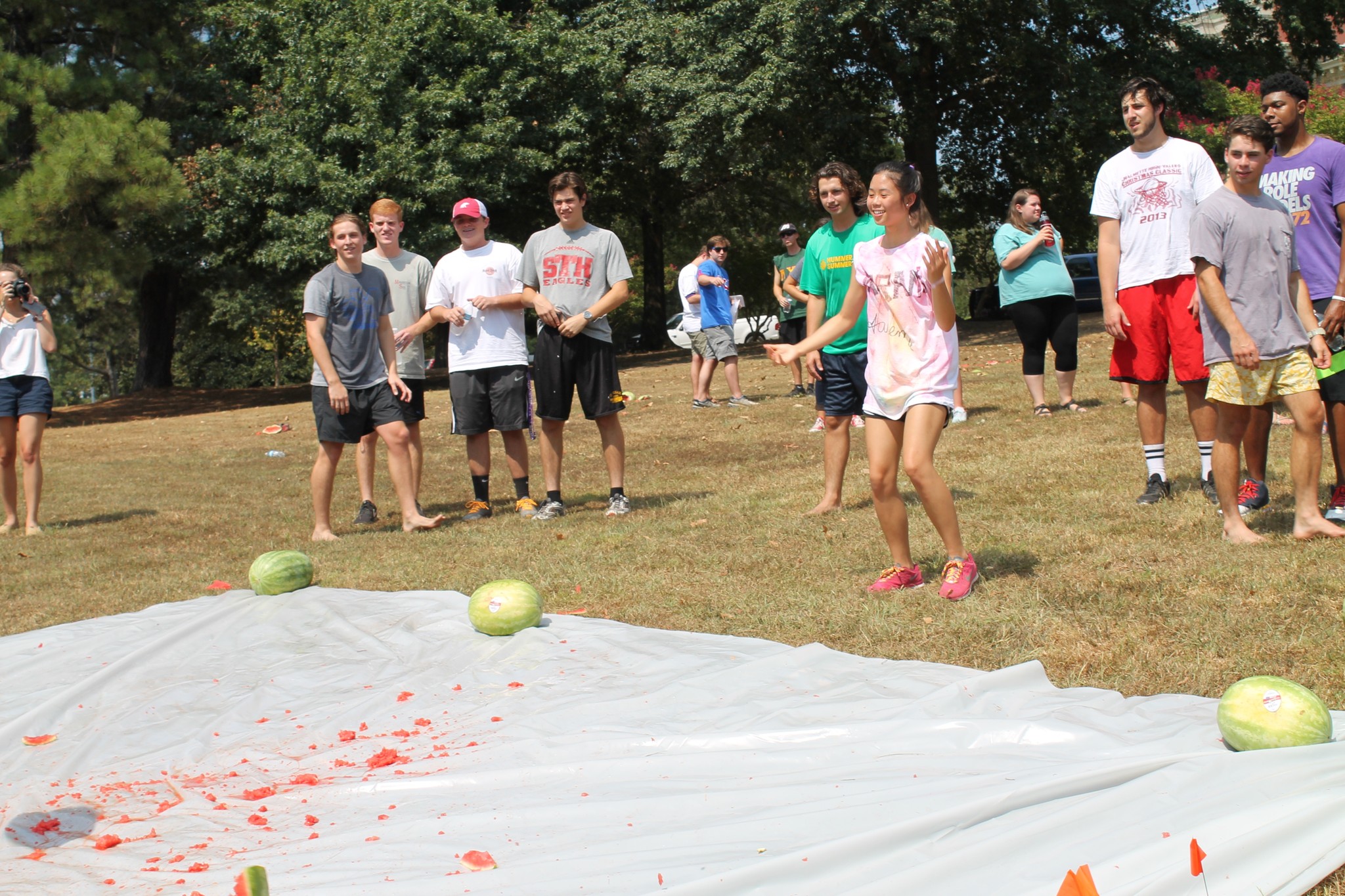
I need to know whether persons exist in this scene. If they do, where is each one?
[515,173,634,520]
[763,160,981,601]
[991,187,1087,417]
[425,197,538,521]
[677,243,721,402]
[697,234,758,407]
[801,163,886,515]
[1187,114,1345,548]
[783,218,866,433]
[1215,73,1345,524]
[1089,75,1225,505]
[772,223,816,397]
[0,262,58,536]
[354,198,437,526]
[302,212,448,540]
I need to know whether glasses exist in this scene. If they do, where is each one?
[779,230,796,238]
[712,247,728,252]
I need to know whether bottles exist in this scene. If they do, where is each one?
[783,294,792,313]
[1039,211,1054,247]
[264,451,286,458]
[451,301,474,336]
[392,328,403,365]
[1312,309,1345,351]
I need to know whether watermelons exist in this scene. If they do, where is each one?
[402,692,414,696]
[248,814,267,825]
[249,550,313,596]
[366,747,398,768]
[206,579,232,590]
[94,834,122,850]
[339,730,355,741]
[294,774,318,786]
[242,786,275,800]
[390,729,409,737]
[232,865,269,896]
[468,579,543,636]
[397,694,407,701]
[1217,674,1333,751]
[33,818,61,834]
[305,815,318,826]
[460,850,498,870]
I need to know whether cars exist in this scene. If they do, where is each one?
[967,252,1104,322]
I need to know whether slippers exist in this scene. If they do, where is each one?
[1122,398,1137,407]
[1034,403,1052,418]
[1057,399,1087,413]
[24,526,41,536]
[0,525,19,535]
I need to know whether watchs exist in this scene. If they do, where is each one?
[582,310,594,322]
[33,314,44,321]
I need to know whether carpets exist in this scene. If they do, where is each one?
[0,586,1345,896]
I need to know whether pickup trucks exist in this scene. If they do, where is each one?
[632,309,781,353]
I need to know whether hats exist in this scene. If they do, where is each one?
[451,197,488,222]
[776,223,797,235]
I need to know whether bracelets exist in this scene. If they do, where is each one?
[930,276,945,289]
[1332,295,1345,301]
[1307,328,1326,338]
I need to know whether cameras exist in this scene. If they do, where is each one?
[10,279,30,298]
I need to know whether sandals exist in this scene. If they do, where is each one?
[1321,421,1328,436]
[708,396,725,404]
[1273,412,1295,425]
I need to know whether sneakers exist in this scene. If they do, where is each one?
[810,416,825,432]
[415,499,423,516]
[605,493,630,518]
[531,498,565,521]
[1217,478,1270,517]
[868,563,924,594]
[939,552,978,600]
[462,499,492,521]
[1325,484,1345,520]
[1136,473,1171,505]
[850,415,865,427]
[1200,470,1220,507]
[515,496,538,518]
[353,500,377,525]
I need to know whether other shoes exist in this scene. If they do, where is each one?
[952,406,967,422]
[807,386,815,395]
[784,388,805,397]
[728,394,759,406]
[691,398,721,409]
